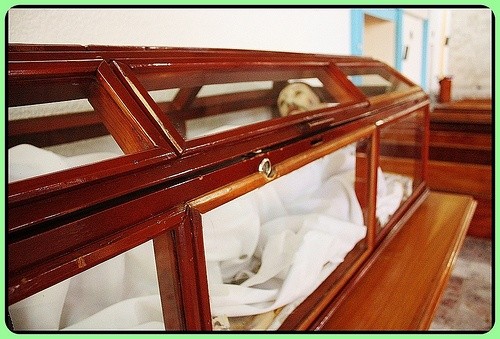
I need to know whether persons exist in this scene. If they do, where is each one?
[10,78,406,331]
